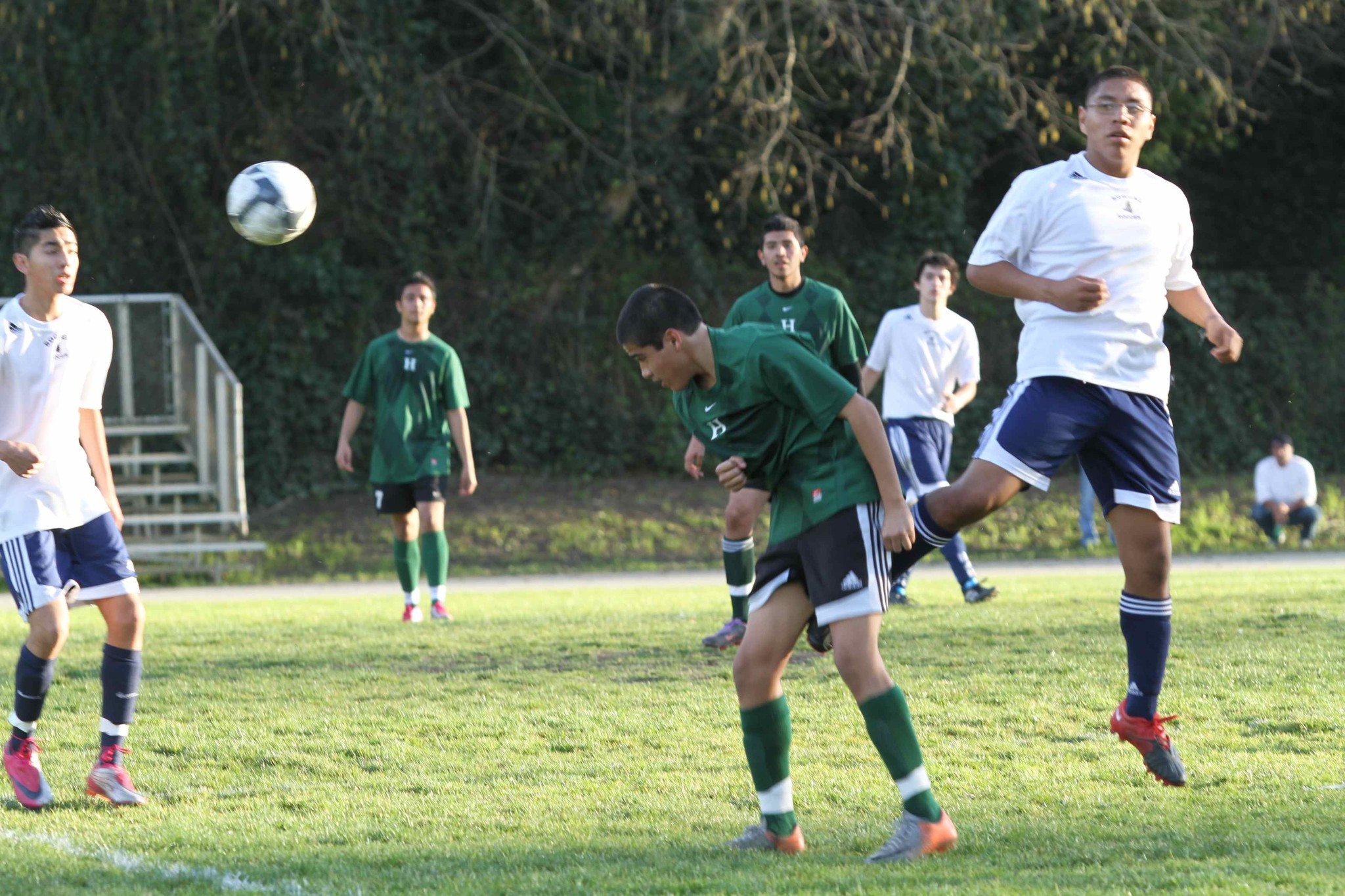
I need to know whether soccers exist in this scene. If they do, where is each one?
[226,159,318,245]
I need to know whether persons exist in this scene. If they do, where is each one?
[684,215,871,651]
[861,253,998,606]
[806,66,1243,786]
[335,272,478,621]
[616,284,957,864]
[1,208,146,811]
[1251,434,1320,551]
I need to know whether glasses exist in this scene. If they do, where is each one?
[1083,101,1152,118]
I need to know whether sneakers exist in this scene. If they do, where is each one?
[1109,698,1185,787]
[867,806,957,862]
[3,734,55,807]
[807,619,834,653]
[432,601,450,619]
[726,819,806,853]
[701,619,746,648]
[85,746,149,807]
[403,605,422,623]
[890,578,908,604]
[964,580,996,602]
